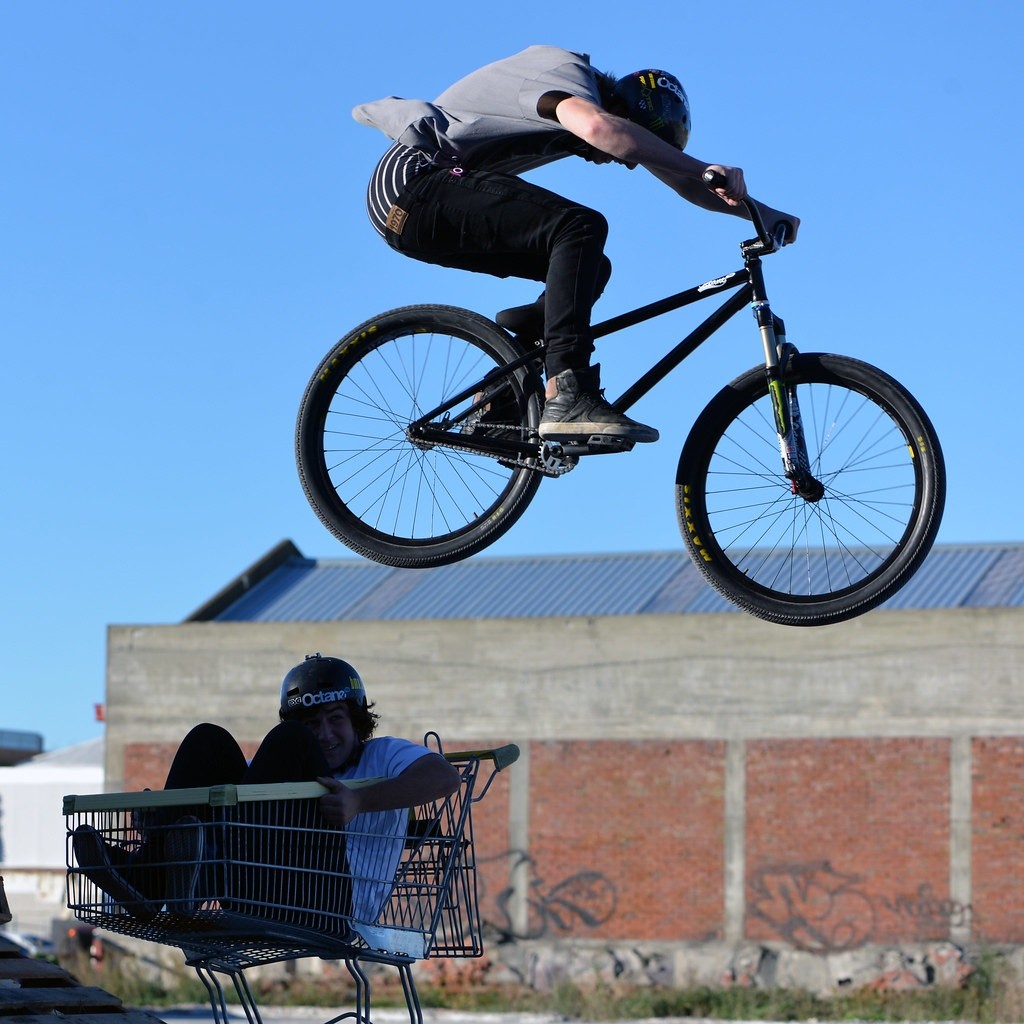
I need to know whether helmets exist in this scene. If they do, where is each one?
[279,654,368,710]
[614,68,692,151]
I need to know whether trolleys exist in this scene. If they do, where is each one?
[63,730,522,1024]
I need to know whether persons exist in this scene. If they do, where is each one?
[348,47,801,442]
[70,652,460,933]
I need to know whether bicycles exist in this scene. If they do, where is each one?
[292,168,947,629]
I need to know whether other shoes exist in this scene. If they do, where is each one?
[459,404,522,441]
[538,362,659,442]
[162,814,204,912]
[73,824,167,920]
